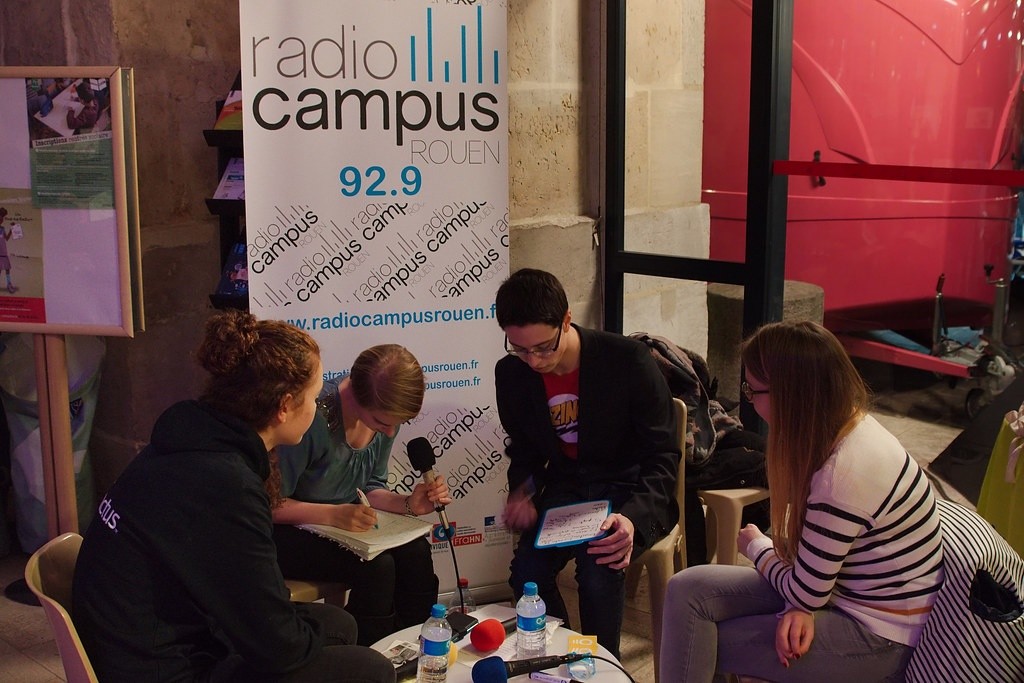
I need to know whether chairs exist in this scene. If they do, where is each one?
[23,394,1024,683]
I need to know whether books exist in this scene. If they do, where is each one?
[295,506,434,561]
[215,243,248,295]
[214,90,244,130]
[212,158,244,199]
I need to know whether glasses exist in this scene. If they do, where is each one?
[742,381,773,401]
[503,325,562,359]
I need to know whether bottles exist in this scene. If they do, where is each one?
[449,578,477,615]
[514,582,548,660]
[415,603,453,683]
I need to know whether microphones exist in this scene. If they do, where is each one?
[472,653,586,683]
[470,617,519,652]
[407,437,452,540]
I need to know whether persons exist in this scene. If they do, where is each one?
[0,207,16,294]
[273,344,453,646]
[28,81,109,135]
[494,269,679,659]
[658,321,944,683]
[69,312,397,683]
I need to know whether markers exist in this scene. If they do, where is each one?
[356,488,378,529]
[529,672,584,683]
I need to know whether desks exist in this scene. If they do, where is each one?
[699,485,770,567]
[370,611,630,683]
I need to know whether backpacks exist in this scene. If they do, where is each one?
[628,331,743,468]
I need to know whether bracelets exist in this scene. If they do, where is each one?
[403,495,418,517]
